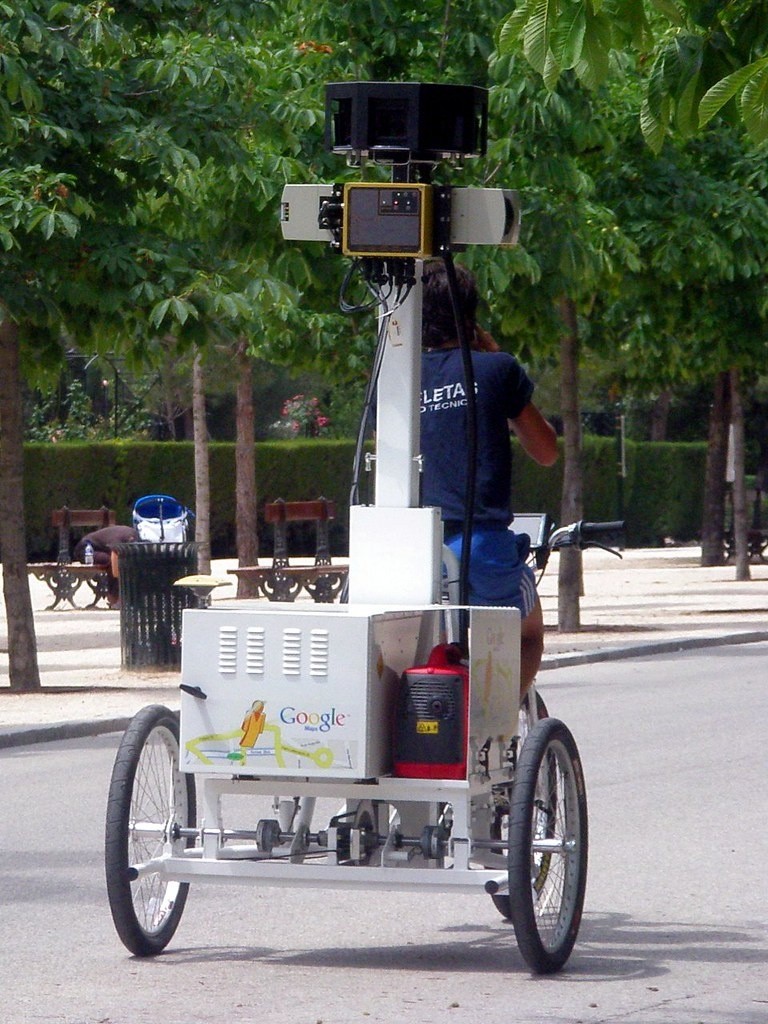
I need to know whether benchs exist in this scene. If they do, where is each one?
[719,487,768,564]
[226,494,351,605]
[21,505,125,610]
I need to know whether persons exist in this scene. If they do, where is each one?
[369,263,560,702]
[76,525,152,609]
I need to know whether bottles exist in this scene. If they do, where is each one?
[84,540,94,565]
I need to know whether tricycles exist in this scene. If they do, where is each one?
[102,517,626,977]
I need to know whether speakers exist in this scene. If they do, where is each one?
[391,642,472,782]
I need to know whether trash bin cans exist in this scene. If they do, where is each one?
[105,541,207,674]
